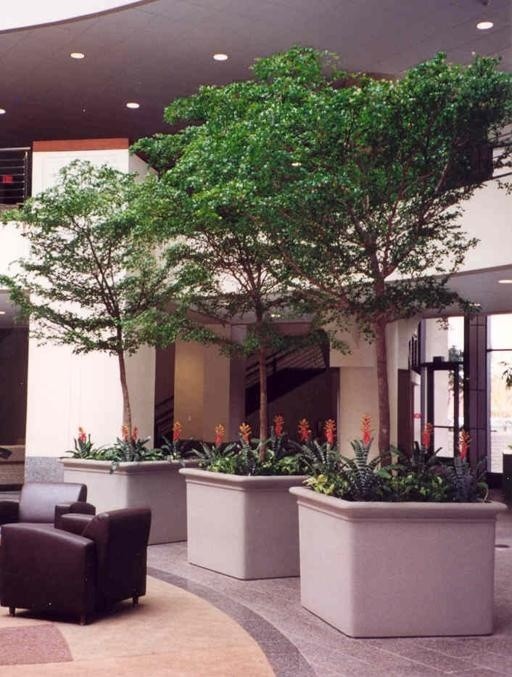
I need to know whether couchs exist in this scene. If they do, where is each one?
[1,477,98,540]
[2,504,161,629]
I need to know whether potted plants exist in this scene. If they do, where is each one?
[0,151,242,551]
[161,58,512,639]
[139,55,345,585]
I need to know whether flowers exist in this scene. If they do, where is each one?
[102,423,149,459]
[158,414,193,460]
[67,416,101,459]
[333,410,384,499]
[432,422,489,502]
[195,413,233,477]
[305,414,342,502]
[259,408,287,476]
[396,420,436,501]
[292,414,313,474]
[229,418,258,474]
